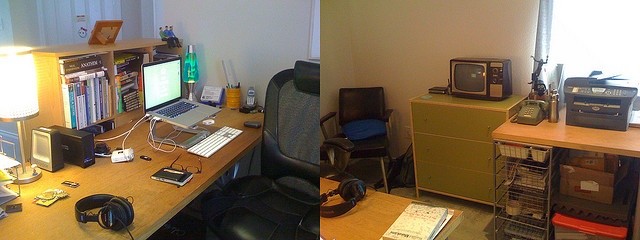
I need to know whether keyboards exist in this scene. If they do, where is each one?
[186,126,244,159]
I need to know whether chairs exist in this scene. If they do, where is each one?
[320,86,393,194]
[200,60,320,239]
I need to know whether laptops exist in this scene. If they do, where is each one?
[140,56,222,129]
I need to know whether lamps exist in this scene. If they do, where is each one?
[0,46,42,184]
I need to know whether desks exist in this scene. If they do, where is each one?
[490,103,640,240]
[320,177,465,240]
[0,103,265,240]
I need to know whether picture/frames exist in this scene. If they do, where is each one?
[87,20,123,45]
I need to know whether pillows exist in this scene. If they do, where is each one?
[341,118,387,140]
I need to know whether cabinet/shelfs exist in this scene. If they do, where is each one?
[32,39,183,137]
[409,92,528,209]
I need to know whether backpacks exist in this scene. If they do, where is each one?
[397,143,415,188]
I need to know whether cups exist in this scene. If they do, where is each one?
[225,87,242,110]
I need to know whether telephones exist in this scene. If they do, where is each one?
[510,99,548,126]
[247,82,257,109]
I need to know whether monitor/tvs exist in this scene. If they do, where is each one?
[450,57,513,101]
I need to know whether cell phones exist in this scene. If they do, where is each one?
[244,121,261,129]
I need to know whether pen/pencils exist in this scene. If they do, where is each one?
[231,84,233,88]
[238,82,240,88]
[228,83,230,88]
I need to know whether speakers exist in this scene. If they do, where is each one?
[48,124,95,169]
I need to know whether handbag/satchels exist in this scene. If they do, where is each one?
[374,159,401,189]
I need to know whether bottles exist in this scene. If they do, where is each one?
[549,94,558,123]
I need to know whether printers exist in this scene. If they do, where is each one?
[564,71,638,132]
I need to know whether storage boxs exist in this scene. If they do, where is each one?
[559,148,630,204]
[552,210,630,240]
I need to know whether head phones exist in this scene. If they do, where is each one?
[320,178,366,218]
[75,194,135,230]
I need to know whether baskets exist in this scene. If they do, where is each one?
[497,215,547,239]
[498,160,547,189]
[496,143,550,164]
[499,189,546,214]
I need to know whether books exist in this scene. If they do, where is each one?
[379,200,454,240]
[59,53,114,130]
[114,54,149,113]
[150,166,193,187]
